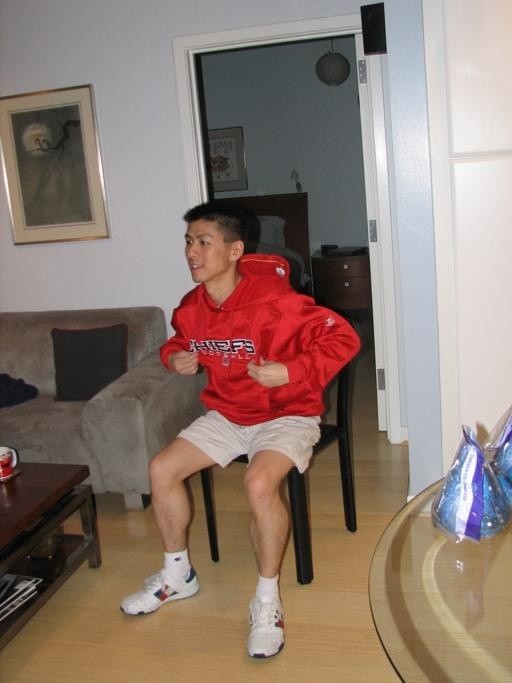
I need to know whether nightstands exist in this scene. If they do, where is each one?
[311,248,374,319]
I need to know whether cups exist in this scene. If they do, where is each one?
[0,446,18,482]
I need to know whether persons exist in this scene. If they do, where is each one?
[118,200,363,659]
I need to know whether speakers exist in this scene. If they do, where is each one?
[360,2,386,55]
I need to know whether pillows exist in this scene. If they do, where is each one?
[240,216,287,253]
[50,324,132,403]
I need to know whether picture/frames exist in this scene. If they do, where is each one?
[0,84,109,246]
[205,125,249,192]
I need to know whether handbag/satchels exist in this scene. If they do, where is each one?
[431,406,512,544]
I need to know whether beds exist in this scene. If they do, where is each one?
[210,193,313,298]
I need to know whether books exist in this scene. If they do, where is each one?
[0,571,44,625]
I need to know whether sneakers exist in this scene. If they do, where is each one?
[247,598,284,657]
[120,565,199,615]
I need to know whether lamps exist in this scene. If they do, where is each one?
[315,38,350,87]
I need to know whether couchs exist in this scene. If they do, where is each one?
[0,307,216,510]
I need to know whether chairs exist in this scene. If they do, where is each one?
[194,306,357,584]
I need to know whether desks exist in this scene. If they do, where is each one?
[368,470,512,683]
